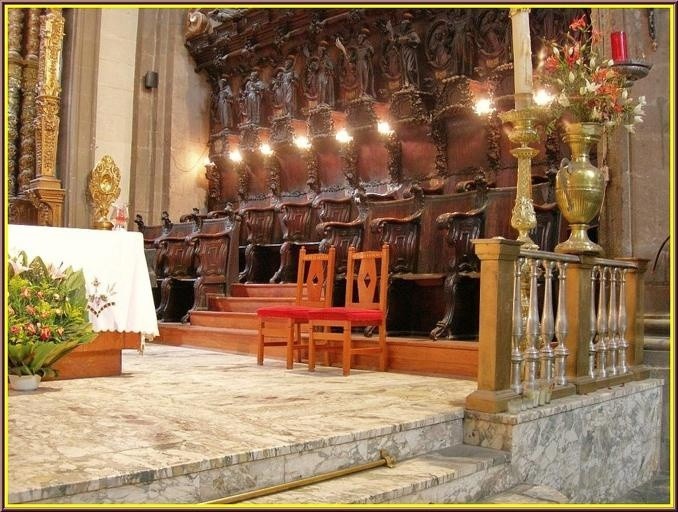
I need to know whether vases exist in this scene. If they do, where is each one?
[8,373,41,391]
[554,121,607,255]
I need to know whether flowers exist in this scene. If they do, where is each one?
[534,14,648,135]
[8,250,98,375]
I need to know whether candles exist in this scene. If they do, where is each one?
[610,31,627,61]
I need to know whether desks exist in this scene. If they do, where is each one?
[8,224,161,381]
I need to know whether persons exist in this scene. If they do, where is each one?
[214,8,587,133]
